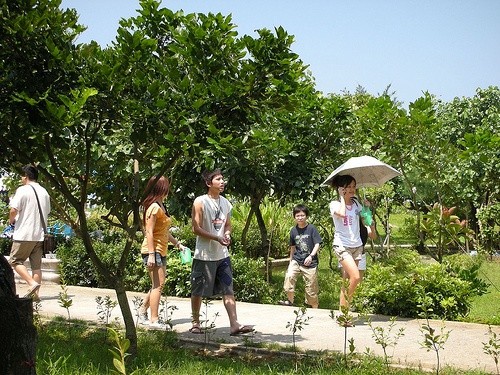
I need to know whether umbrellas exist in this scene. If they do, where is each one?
[319,154,401,205]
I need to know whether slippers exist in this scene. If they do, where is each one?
[230,326,254,336]
[189,326,203,334]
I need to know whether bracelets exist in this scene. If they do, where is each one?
[175,241,180,247]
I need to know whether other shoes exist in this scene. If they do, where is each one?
[137,312,151,325]
[150,319,170,329]
[19,281,40,299]
[278,300,294,306]
[337,317,354,326]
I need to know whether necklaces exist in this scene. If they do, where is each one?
[209,194,220,209]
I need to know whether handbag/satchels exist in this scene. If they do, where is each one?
[352,199,368,246]
[44,234,57,252]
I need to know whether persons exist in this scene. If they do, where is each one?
[328,175,376,328]
[140,174,184,330]
[189,170,256,336]
[279,204,322,308]
[8,165,51,301]
